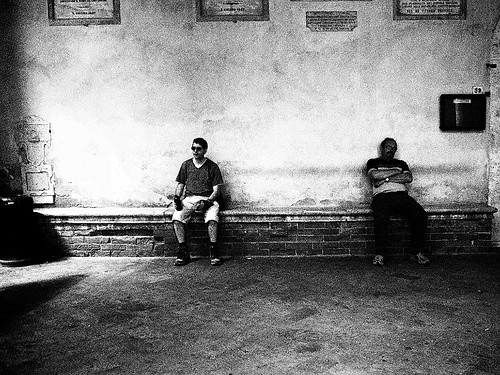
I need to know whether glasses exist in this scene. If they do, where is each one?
[191,147,204,151]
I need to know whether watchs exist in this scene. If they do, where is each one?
[385,177,390,182]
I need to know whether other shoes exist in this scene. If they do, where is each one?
[174,247,191,265]
[209,245,221,265]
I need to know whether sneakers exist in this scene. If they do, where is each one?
[411,253,429,264]
[373,255,384,266]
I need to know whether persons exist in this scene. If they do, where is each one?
[366,138,430,265]
[171,138,225,265]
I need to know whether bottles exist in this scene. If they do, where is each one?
[174,195,183,211]
